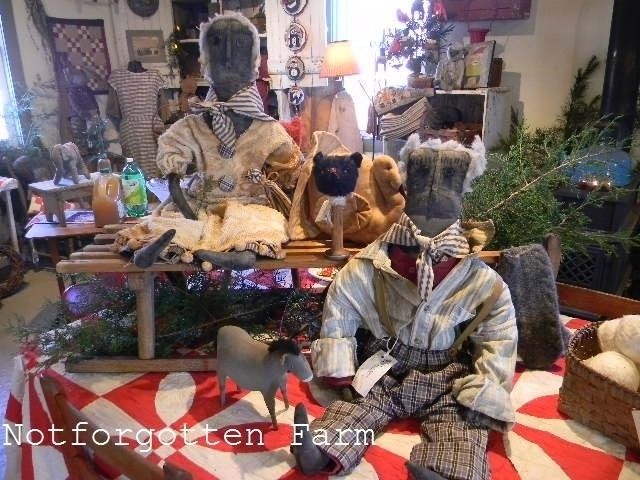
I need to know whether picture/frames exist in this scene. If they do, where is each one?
[125,29,167,64]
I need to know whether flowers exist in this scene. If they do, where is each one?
[366,0,457,77]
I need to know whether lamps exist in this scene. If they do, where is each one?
[318,38,364,87]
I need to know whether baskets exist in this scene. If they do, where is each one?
[1,244,26,298]
[556,319,640,451]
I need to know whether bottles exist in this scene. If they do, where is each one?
[91,157,148,229]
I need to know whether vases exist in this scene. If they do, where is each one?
[406,74,434,90]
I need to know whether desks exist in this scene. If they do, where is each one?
[0,175,22,256]
[1,190,637,480]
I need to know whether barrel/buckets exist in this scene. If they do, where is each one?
[91,157,125,226]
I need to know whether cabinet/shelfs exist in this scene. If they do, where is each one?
[381,87,513,165]
[170,0,269,82]
